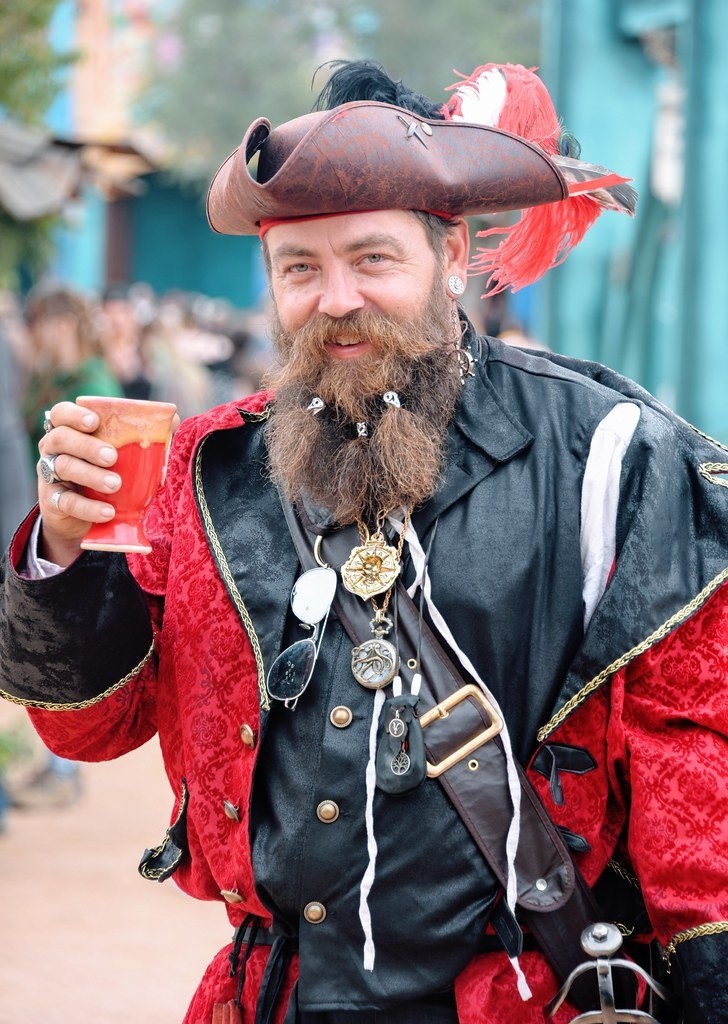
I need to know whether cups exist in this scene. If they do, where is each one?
[74,394,178,553]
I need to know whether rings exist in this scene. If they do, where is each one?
[43,410,55,433]
[50,488,71,512]
[35,454,61,486]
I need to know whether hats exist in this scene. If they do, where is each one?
[205,61,637,299]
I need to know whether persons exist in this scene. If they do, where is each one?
[0,59,727,1023]
[0,277,279,811]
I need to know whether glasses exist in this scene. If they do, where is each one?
[267,567,337,709]
